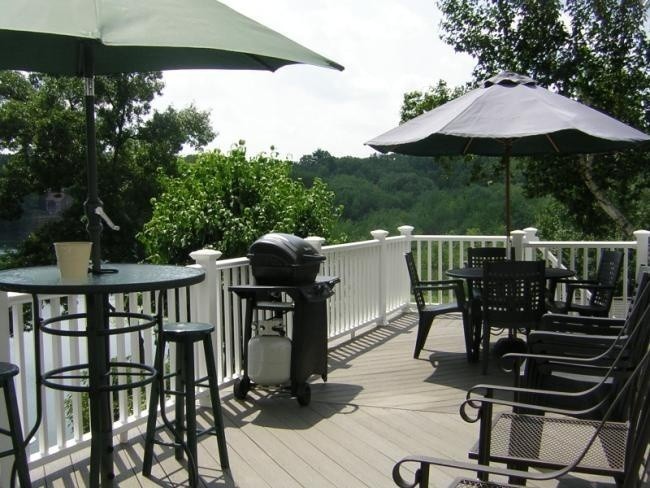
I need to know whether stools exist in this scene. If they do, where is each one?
[0,362,32,488]
[142,321,230,487]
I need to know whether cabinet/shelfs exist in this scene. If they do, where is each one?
[227,276,341,407]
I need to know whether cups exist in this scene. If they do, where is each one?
[53,241,93,282]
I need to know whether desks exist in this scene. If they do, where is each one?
[0,265,205,488]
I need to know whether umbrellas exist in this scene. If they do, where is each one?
[363,70,650,260]
[0,0,341,276]
